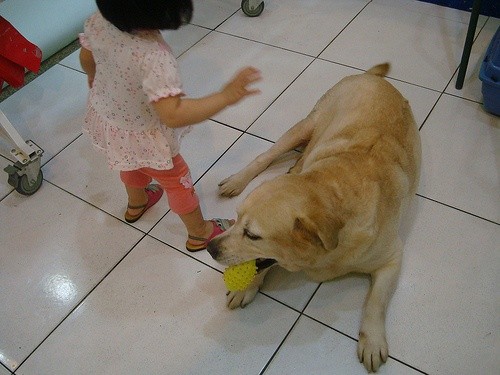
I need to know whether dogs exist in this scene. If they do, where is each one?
[219,62,423,372]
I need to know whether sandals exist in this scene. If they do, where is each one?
[186,217,236,253]
[125,184,164,223]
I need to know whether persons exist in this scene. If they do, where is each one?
[78,0,262,252]
